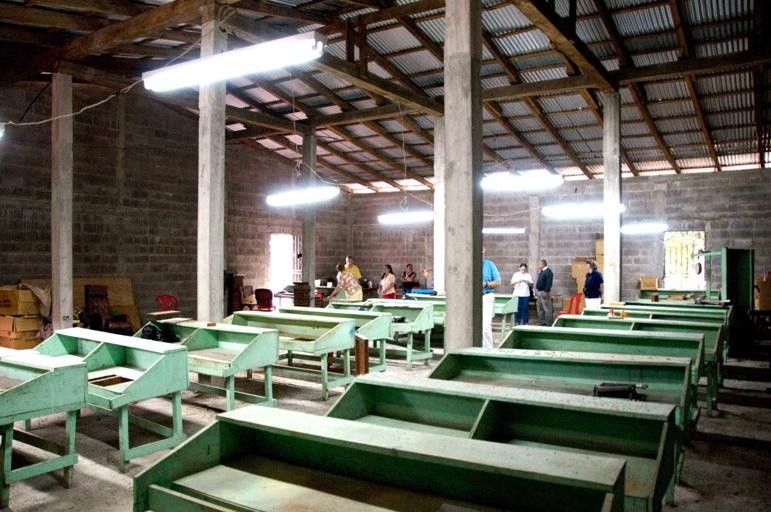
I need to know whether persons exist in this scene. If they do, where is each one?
[511,264,534,326]
[583,261,604,309]
[400,264,417,291]
[536,259,554,326]
[482,248,501,349]
[379,264,396,299]
[327,264,363,300]
[344,256,361,281]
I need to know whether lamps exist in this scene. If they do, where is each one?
[141,30,329,93]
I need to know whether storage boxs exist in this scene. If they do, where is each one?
[640,277,659,290]
[562,238,603,314]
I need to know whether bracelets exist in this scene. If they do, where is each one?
[486,281,490,288]
